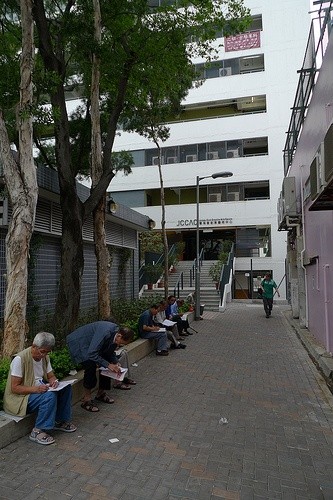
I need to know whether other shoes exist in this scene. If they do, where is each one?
[156,350,168,356]
[179,333,189,336]
[269,310,272,314]
[177,336,184,340]
[176,343,184,349]
[187,332,193,335]
[266,314,269,318]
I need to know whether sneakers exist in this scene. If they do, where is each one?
[52,420,76,432]
[29,428,55,444]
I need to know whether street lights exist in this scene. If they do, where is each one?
[195,171,234,320]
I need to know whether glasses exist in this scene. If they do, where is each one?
[36,348,52,356]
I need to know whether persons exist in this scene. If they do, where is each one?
[137,304,169,355]
[2,331,77,444]
[165,296,185,340]
[171,298,193,336]
[152,301,188,349]
[67,321,134,413]
[260,272,281,318]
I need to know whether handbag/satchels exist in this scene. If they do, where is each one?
[258,288,263,295]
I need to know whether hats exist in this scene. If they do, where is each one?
[179,299,185,304]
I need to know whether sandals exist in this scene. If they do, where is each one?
[124,376,136,385]
[113,382,131,389]
[81,399,99,412]
[95,392,115,403]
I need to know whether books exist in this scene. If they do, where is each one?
[162,319,177,326]
[99,366,129,381]
[152,328,166,332]
[42,379,79,391]
[180,312,192,321]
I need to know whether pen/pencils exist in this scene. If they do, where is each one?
[39,380,50,390]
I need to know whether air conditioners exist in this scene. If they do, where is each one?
[208,193,221,202]
[167,157,177,163]
[186,155,197,162]
[228,192,239,201]
[152,156,164,165]
[218,67,232,76]
[277,177,296,227]
[226,149,238,158]
[206,151,218,159]
[310,121,333,199]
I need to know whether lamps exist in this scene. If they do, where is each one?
[106,193,117,214]
[147,216,155,230]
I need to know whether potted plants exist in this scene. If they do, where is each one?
[208,262,224,290]
[142,264,162,290]
[169,253,181,272]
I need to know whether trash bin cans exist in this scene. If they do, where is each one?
[200,304,205,315]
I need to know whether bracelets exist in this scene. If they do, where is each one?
[278,293,279,294]
[55,380,59,382]
